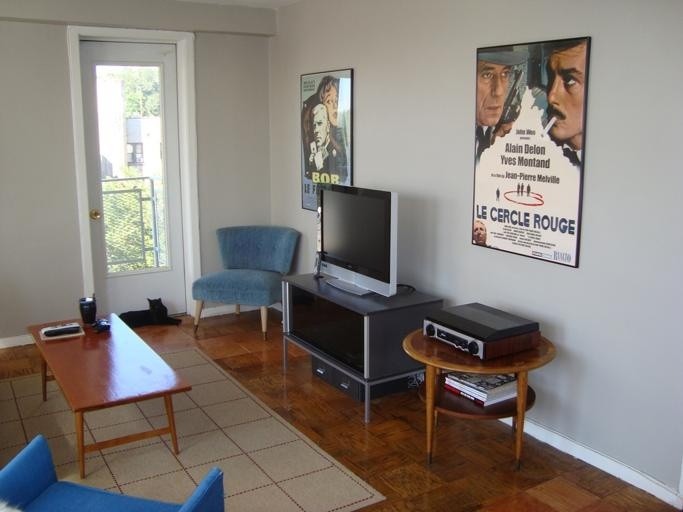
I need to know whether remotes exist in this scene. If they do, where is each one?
[44,326,81,337]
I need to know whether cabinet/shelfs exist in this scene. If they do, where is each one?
[277,272,443,425]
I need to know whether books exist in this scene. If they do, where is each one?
[444,371,519,408]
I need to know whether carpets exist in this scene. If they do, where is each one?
[0,343,388,511]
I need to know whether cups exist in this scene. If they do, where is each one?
[78,297,96,323]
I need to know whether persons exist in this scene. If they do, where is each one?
[543,38,589,166]
[317,76,345,147]
[472,220,486,246]
[475,46,530,162]
[304,102,347,183]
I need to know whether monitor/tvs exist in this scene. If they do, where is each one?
[316,182,399,298]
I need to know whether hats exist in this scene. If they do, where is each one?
[476,45,532,70]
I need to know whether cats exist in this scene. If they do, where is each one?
[118,298,182,329]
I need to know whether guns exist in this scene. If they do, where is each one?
[494,68,524,135]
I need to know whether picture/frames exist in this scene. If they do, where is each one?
[298,67,354,212]
[471,33,592,269]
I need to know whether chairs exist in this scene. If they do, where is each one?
[191,223,301,342]
[0,433,226,511]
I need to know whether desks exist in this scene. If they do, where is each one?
[401,329,556,469]
[27,311,191,479]
